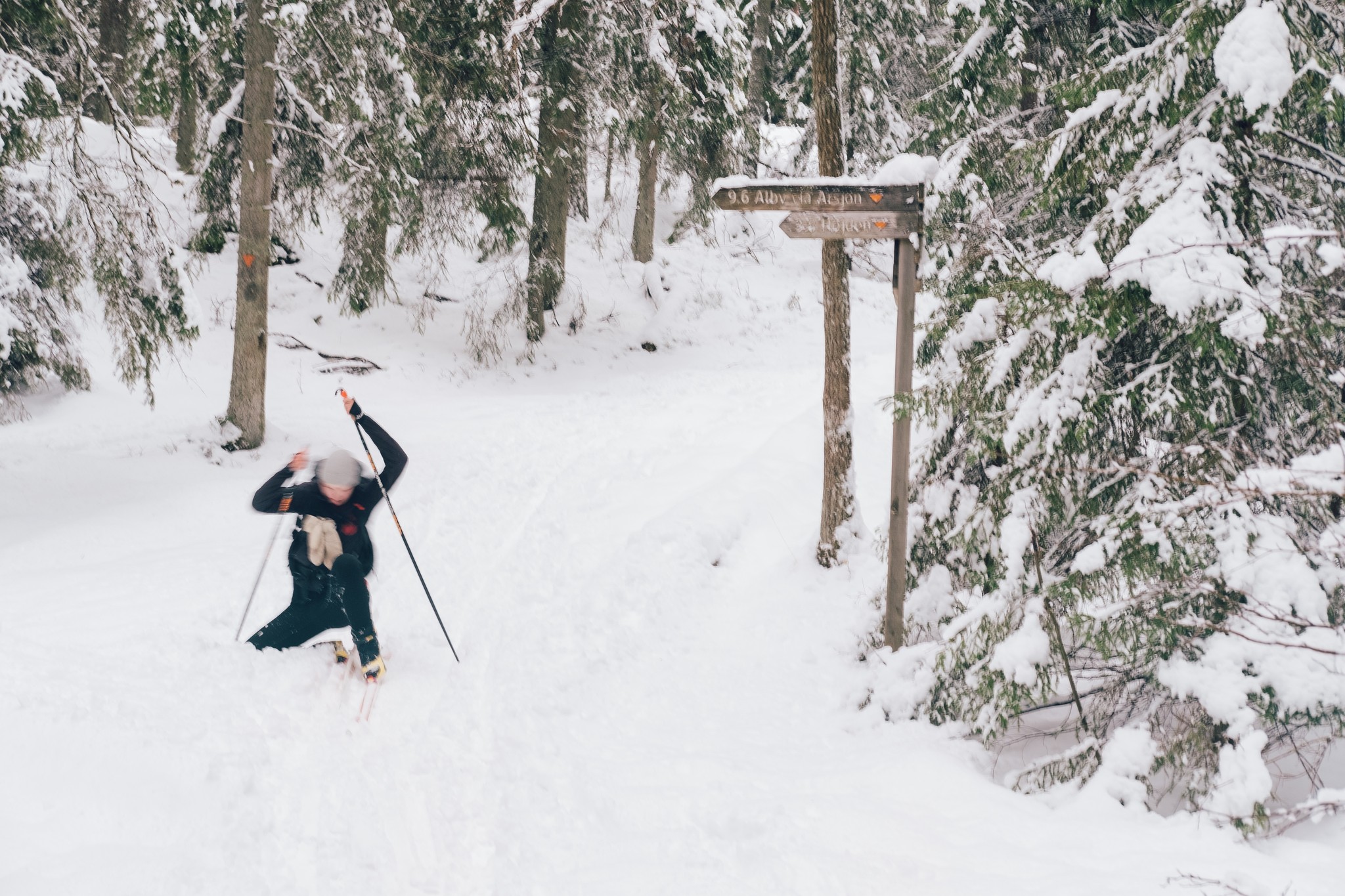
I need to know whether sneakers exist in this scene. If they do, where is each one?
[349,623,380,679]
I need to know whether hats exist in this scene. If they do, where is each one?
[321,450,361,487]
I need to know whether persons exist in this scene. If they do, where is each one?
[246,397,408,682]
